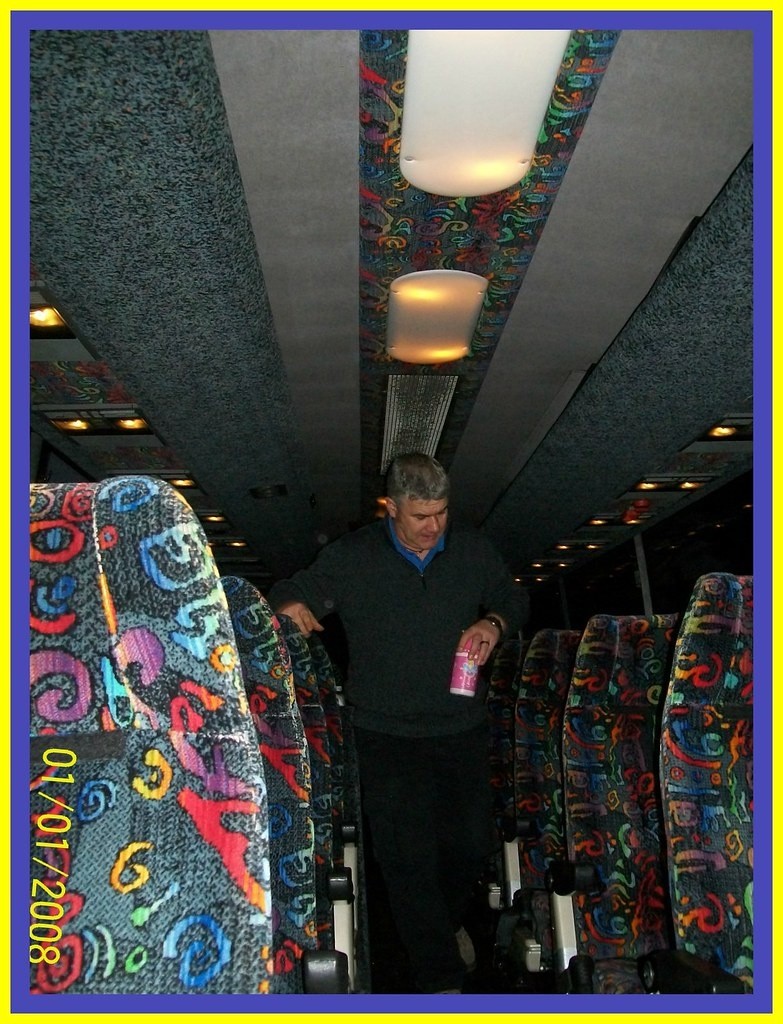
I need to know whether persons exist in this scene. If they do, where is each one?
[276,451,534,997]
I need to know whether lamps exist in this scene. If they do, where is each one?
[384,269,490,364]
[400,29,573,199]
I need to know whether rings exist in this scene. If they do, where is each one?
[480,641,489,644]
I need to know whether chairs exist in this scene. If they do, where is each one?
[29,473,367,995]
[475,571,753,994]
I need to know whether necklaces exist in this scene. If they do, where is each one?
[404,546,425,558]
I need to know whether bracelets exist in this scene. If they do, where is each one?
[483,616,503,638]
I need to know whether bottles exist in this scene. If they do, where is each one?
[449,640,478,697]
